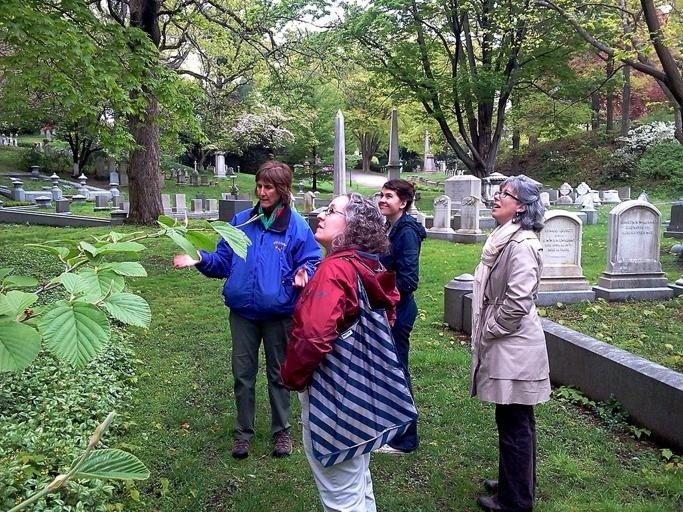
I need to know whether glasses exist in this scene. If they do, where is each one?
[323,208,346,216]
[495,192,523,203]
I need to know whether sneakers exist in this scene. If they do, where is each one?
[372,444,414,456]
[273,430,293,459]
[484,480,499,495]
[232,439,249,460]
[477,494,501,512]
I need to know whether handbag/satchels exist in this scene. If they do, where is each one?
[307,309,418,468]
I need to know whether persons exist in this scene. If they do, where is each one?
[276,191,419,511]
[169,160,326,460]
[373,178,428,454]
[468,175,553,511]
